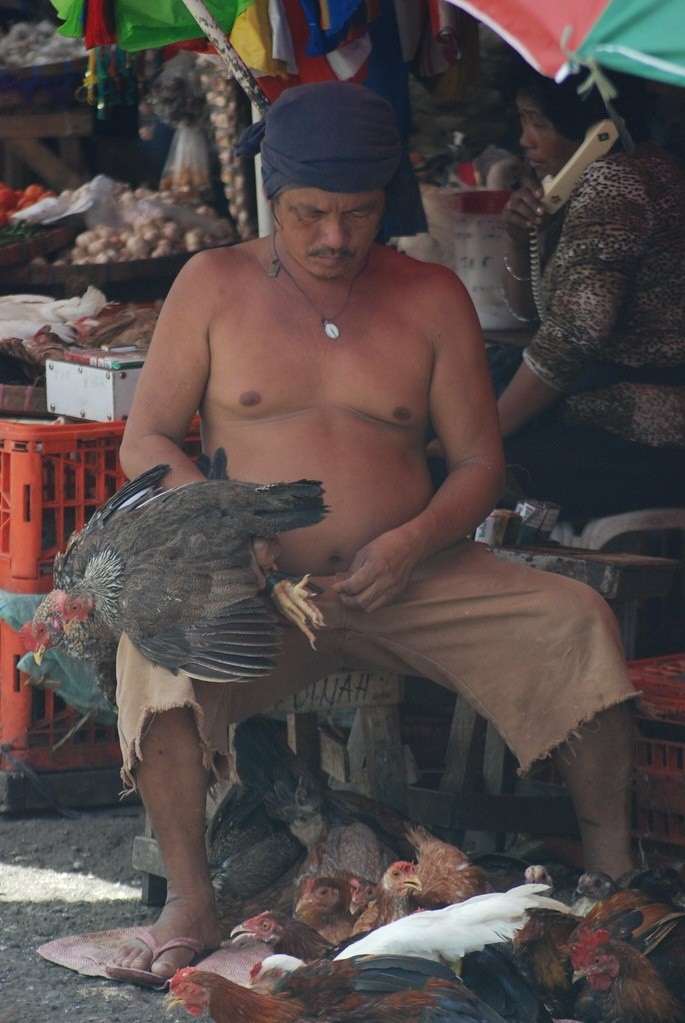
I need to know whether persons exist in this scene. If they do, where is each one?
[425,60,685,535]
[105,80,643,989]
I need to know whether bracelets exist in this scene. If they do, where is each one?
[503,251,530,282]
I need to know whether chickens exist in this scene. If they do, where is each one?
[17,462,334,686]
[159,714,685,1023]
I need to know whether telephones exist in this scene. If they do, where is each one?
[541,117,627,214]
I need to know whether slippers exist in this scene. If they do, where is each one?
[106,932,203,986]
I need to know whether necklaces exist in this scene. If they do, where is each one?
[272,231,371,338]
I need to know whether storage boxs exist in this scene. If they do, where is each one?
[611,655,685,878]
[0,359,202,774]
